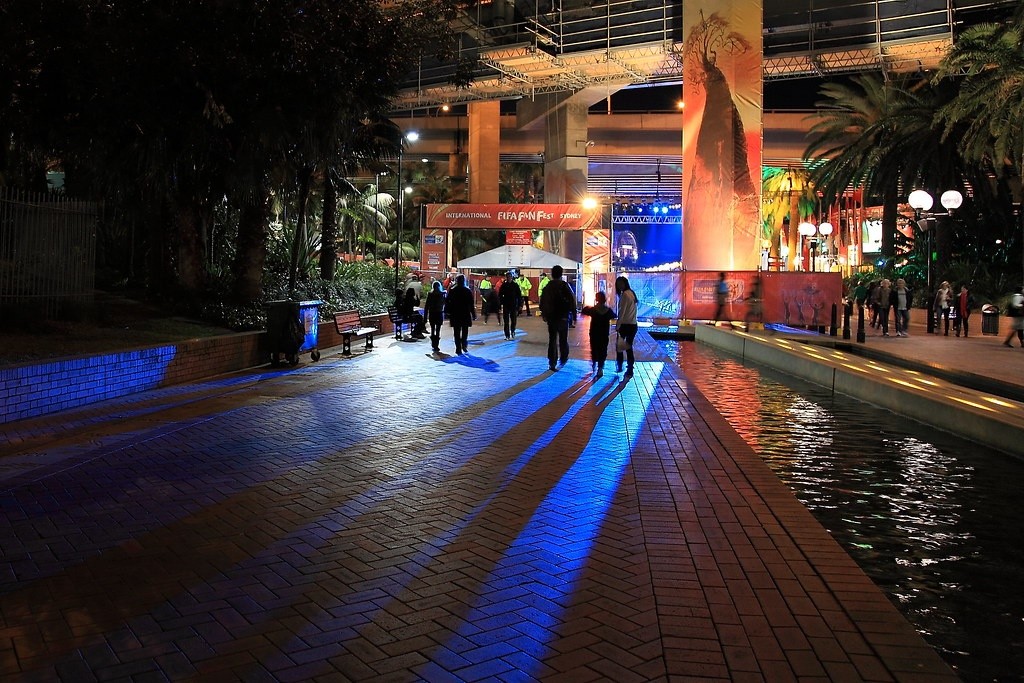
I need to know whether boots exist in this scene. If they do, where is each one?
[617,361,622,372]
[625,366,634,374]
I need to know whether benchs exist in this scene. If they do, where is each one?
[331,309,378,356]
[387,306,416,341]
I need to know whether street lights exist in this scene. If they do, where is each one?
[394,129,420,298]
[399,183,414,269]
[799,222,834,272]
[373,170,390,267]
[908,190,963,334]
[581,197,613,273]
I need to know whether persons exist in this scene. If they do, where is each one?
[479,272,532,341]
[538,265,577,370]
[956,286,975,338]
[581,292,617,376]
[1003,286,1024,348]
[393,288,430,339]
[713,273,771,332]
[614,276,638,375]
[853,278,911,336]
[424,273,476,354]
[933,281,954,336]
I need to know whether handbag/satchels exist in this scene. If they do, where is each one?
[617,332,630,352]
[1006,297,1024,317]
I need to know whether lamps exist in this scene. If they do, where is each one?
[614,199,669,214]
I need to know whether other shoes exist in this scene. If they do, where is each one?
[956,333,960,338]
[456,349,462,356]
[548,362,555,370]
[597,368,603,377]
[883,332,889,336]
[462,346,468,353]
[897,330,906,336]
[1004,342,1014,348]
[561,358,568,363]
[511,332,516,339]
[504,337,509,341]
[423,330,430,334]
[944,332,948,336]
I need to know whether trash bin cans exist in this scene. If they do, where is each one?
[981,304,999,336]
[266,300,323,365]
